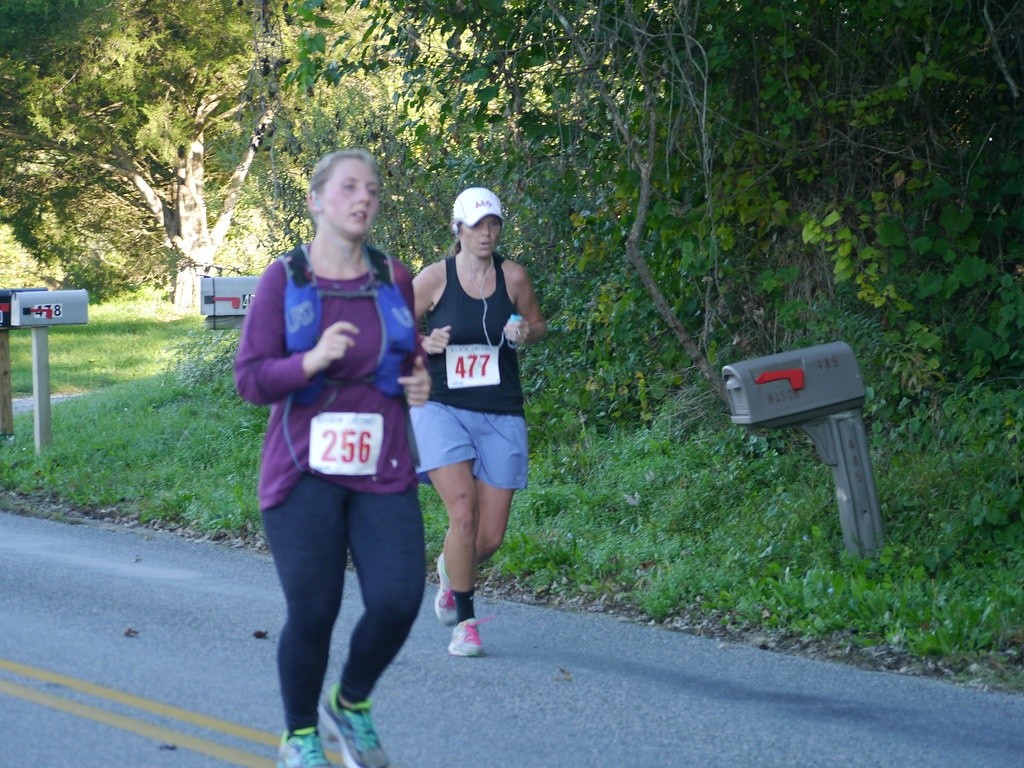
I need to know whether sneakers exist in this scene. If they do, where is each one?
[317,682,390,768]
[434,552,460,625]
[276,722,332,768]
[447,616,493,658]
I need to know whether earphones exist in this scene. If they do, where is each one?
[313,197,319,207]
[452,223,459,236]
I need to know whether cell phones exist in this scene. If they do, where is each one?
[505,314,523,340]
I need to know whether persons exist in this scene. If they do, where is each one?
[408,186,547,657]
[235,148,433,768]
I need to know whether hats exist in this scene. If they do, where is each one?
[453,187,505,228]
